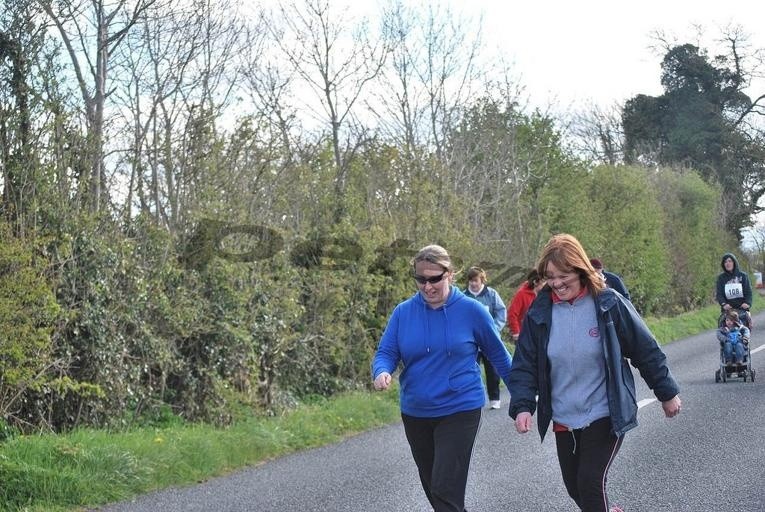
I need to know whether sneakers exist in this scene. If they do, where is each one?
[489,400,501,409]
[727,360,742,366]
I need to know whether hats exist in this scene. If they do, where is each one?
[590,258,603,270]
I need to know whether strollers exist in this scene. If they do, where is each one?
[715,309,757,382]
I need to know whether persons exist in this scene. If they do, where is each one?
[371,245,512,512]
[464,266,506,409]
[507,269,547,342]
[590,259,631,301]
[507,233,682,512]
[716,253,752,377]
[716,310,751,366]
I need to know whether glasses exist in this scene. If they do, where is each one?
[412,271,445,284]
